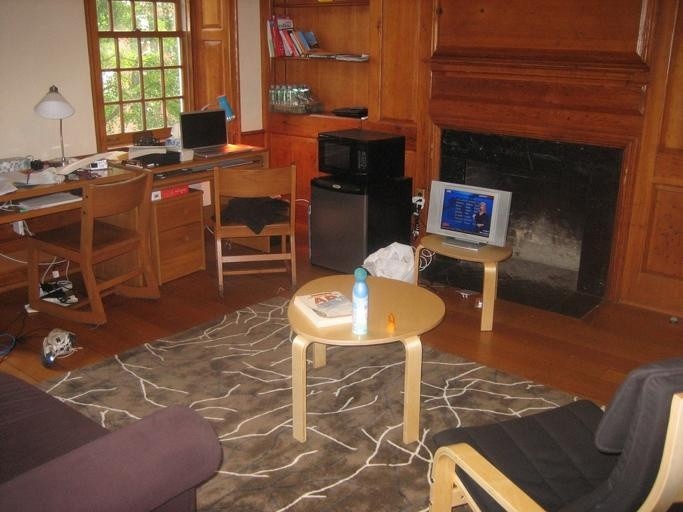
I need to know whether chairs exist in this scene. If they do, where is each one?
[203,159,298,299]
[429,364,683,512]
[25,169,161,327]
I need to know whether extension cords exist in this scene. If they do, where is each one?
[57,295,78,304]
[36,280,73,297]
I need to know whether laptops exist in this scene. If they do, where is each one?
[133,153,181,167]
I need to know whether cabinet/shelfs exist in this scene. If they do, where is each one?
[258,0,425,261]
[146,187,207,286]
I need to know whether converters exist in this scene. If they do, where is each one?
[409,204,417,214]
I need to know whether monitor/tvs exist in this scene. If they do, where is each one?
[179,111,234,158]
[427,180,512,252]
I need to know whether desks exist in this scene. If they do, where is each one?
[0,140,269,298]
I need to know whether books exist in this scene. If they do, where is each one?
[128,146,166,160]
[18,189,87,212]
[267,15,370,62]
[268,79,325,115]
[293,290,353,328]
[150,185,189,204]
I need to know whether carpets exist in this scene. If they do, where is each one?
[34,294,609,510]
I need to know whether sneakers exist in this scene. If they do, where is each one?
[42,327,77,367]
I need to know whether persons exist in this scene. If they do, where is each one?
[476,202,490,231]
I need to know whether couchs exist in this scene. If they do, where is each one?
[0,369,221,510]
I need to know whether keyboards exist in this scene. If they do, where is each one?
[192,158,253,171]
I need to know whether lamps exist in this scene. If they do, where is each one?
[33,85,75,168]
[197,95,237,124]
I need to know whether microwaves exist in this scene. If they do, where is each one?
[316,127,405,184]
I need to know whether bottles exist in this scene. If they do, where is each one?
[351,268,368,335]
[269,83,309,113]
